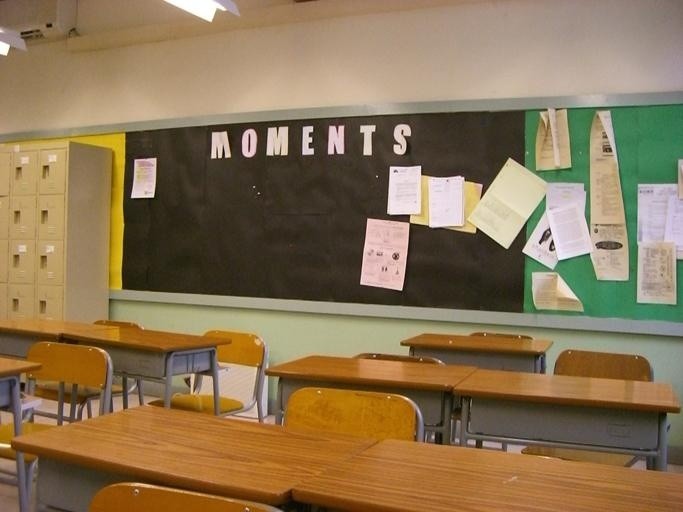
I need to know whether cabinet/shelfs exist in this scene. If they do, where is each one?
[0,139,113,324]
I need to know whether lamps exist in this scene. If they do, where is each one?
[161,0,239,24]
[0,28,28,58]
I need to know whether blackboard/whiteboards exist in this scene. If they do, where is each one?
[1,92,682,337]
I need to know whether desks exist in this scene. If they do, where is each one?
[0,319,683,512]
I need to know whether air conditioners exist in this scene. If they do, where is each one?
[0,0,77,41]
